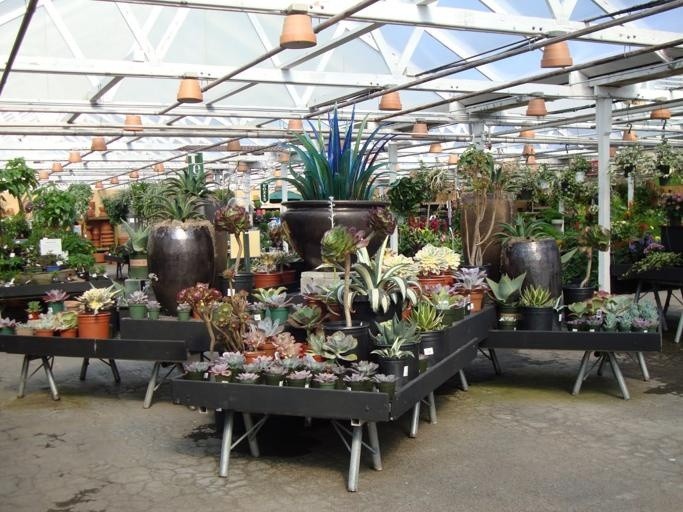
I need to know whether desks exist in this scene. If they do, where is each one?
[168,334,483,495]
